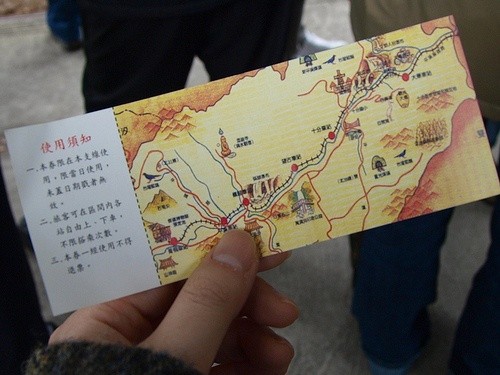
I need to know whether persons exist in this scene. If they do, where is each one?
[43,0,310,115]
[23,228,299,375]
[347,2,500,374]
